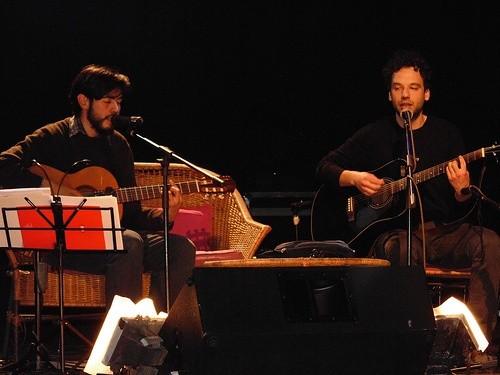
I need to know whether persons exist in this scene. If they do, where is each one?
[0,64,196,314]
[316,58,500,368]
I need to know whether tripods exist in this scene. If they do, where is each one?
[0,186,127,375]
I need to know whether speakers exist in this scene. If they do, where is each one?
[159,264,437,375]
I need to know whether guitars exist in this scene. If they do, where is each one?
[311,143,500,247]
[25,159,236,222]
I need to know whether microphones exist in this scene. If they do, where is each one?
[400,108,413,120]
[111,114,144,124]
[461,185,477,194]
